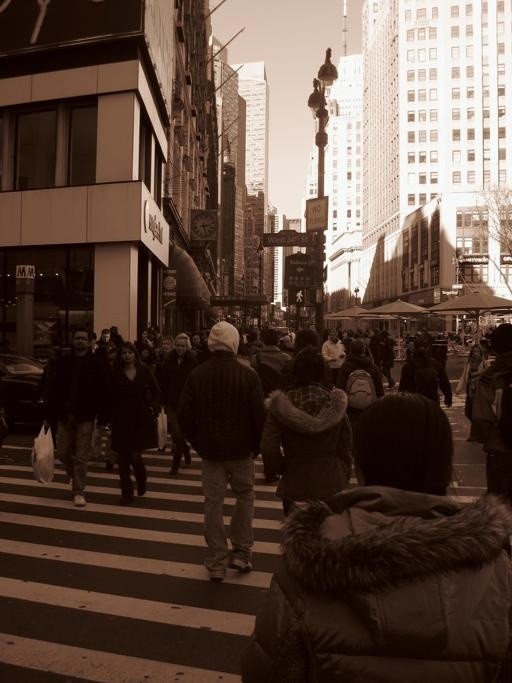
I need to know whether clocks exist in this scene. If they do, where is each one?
[191,211,217,238]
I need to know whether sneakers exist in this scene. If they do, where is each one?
[231,558,252,571]
[69,478,87,505]
[158,444,190,474]
[105,460,114,471]
[137,481,146,495]
[120,496,131,504]
[266,474,279,483]
[210,571,224,580]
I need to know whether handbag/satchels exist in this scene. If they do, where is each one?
[158,414,168,449]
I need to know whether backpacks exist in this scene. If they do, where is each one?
[341,363,378,411]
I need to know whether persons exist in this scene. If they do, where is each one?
[238,392,509,682]
[36,317,512,582]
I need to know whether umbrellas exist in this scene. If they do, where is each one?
[323,306,397,331]
[357,297,429,356]
[426,290,511,342]
[434,309,511,315]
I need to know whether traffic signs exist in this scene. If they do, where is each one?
[262,229,317,247]
[305,196,330,234]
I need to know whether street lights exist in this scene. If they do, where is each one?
[354,287,359,308]
[450,254,466,336]
[308,46,340,343]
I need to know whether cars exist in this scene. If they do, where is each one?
[1,351,56,434]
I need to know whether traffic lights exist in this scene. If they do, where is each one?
[287,286,307,306]
